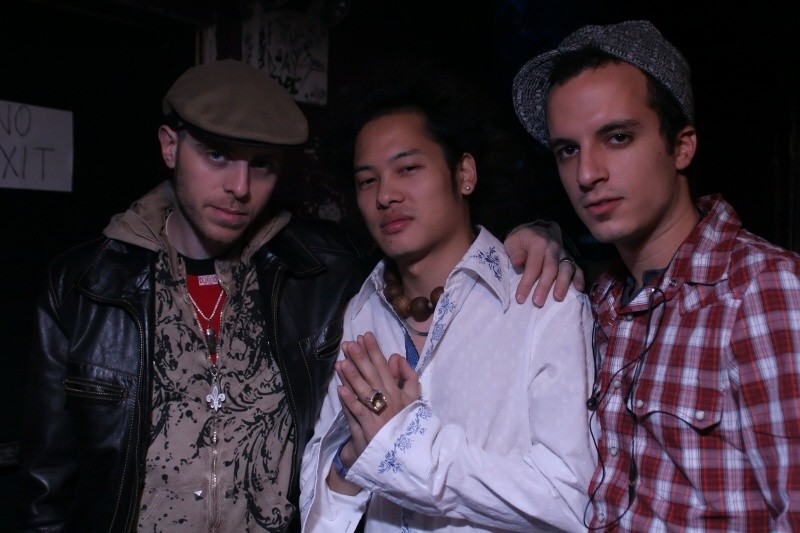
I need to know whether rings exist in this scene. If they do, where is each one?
[559,257,576,268]
[364,388,387,412]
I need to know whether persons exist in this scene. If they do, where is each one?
[0,60,586,533]
[299,81,602,533]
[508,20,800,533]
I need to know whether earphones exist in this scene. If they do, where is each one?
[587,395,596,410]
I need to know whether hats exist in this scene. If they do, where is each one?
[511,19,694,150]
[166,63,308,170]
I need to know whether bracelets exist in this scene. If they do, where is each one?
[334,444,347,478]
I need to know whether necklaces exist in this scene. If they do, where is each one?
[166,212,228,411]
[383,260,444,322]
[404,319,429,335]
